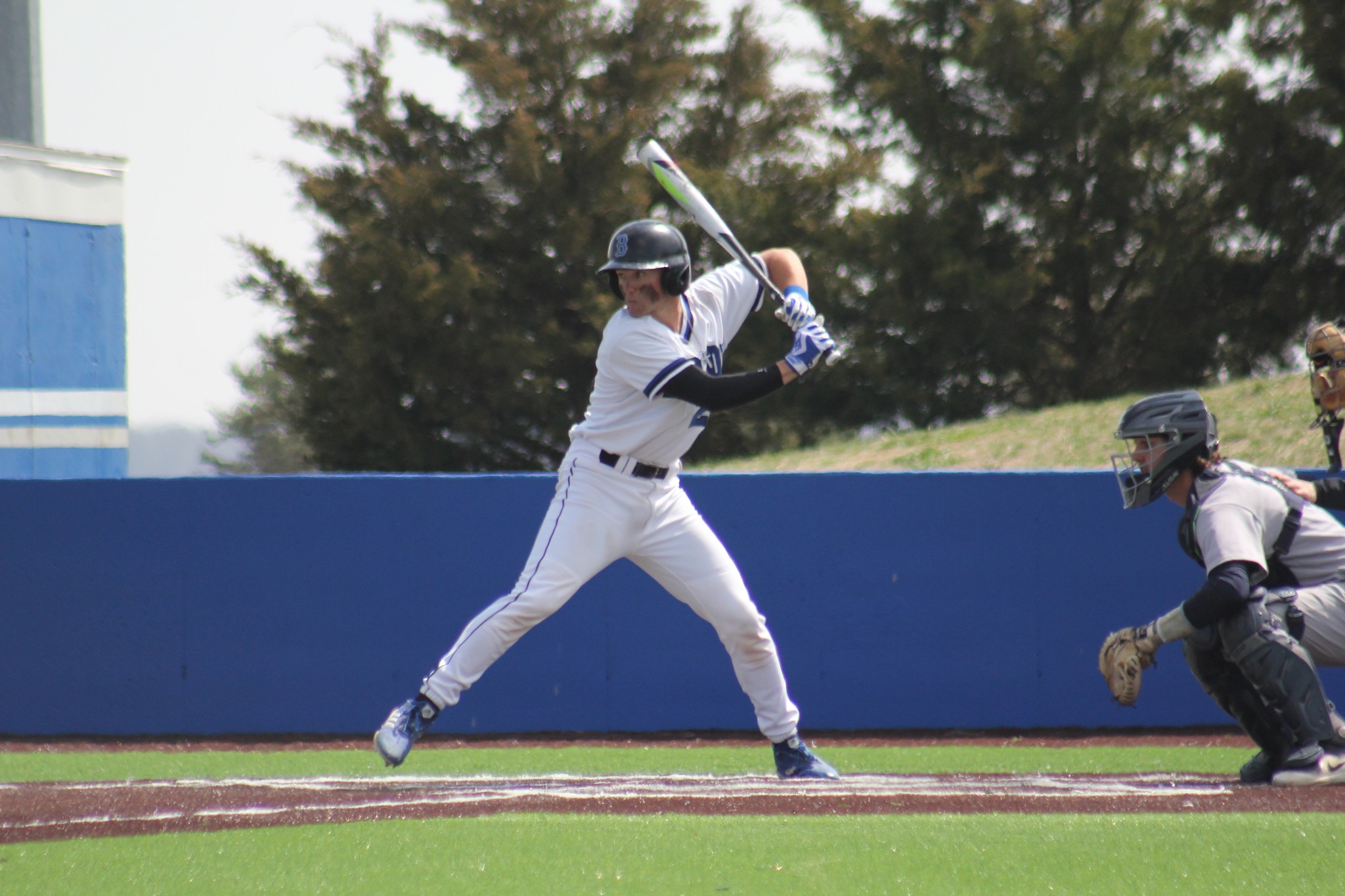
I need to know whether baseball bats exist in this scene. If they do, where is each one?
[635,138,842,366]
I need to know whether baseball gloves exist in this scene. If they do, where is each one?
[1099,618,1165,707]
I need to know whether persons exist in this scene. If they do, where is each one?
[375,222,842,780]
[1098,319,1345,786]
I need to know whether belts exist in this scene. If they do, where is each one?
[599,450,669,480]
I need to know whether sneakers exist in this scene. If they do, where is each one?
[373,701,438,766]
[773,735,839,781]
[1272,754,1345,786]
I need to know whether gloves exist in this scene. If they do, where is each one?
[785,314,834,376]
[775,286,816,330]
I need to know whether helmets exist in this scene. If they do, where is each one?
[595,219,690,300]
[1111,390,1218,510]
